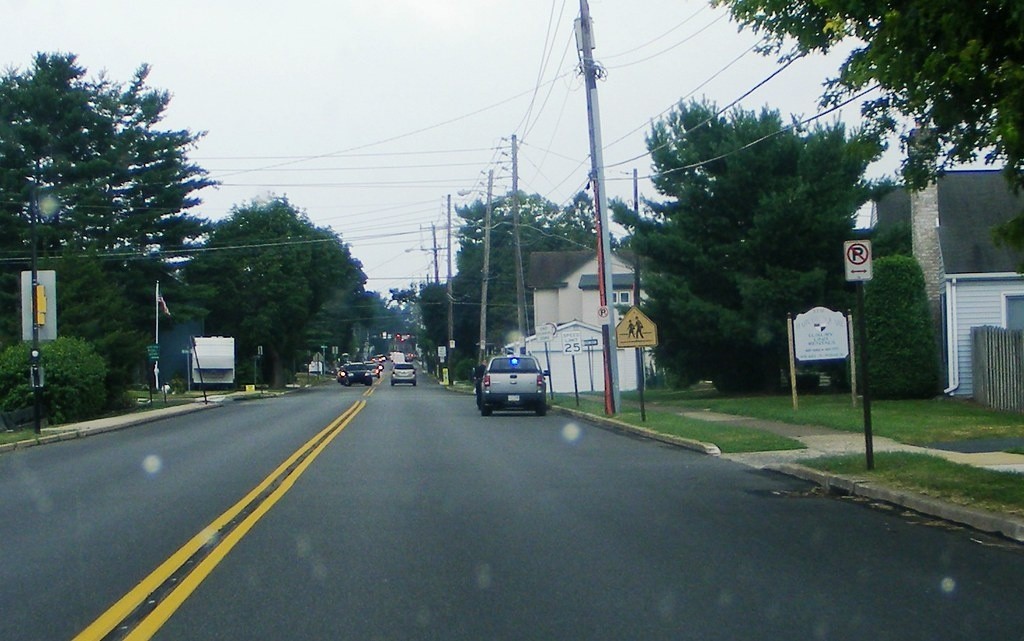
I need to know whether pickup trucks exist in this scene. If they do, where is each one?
[475,353,552,417]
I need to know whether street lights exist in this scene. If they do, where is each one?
[455,188,529,341]
[404,247,454,385]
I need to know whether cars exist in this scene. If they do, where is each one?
[390,362,417,386]
[336,355,387,386]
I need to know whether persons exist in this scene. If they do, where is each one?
[473,360,487,394]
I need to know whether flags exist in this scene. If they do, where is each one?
[158,291,170,315]
